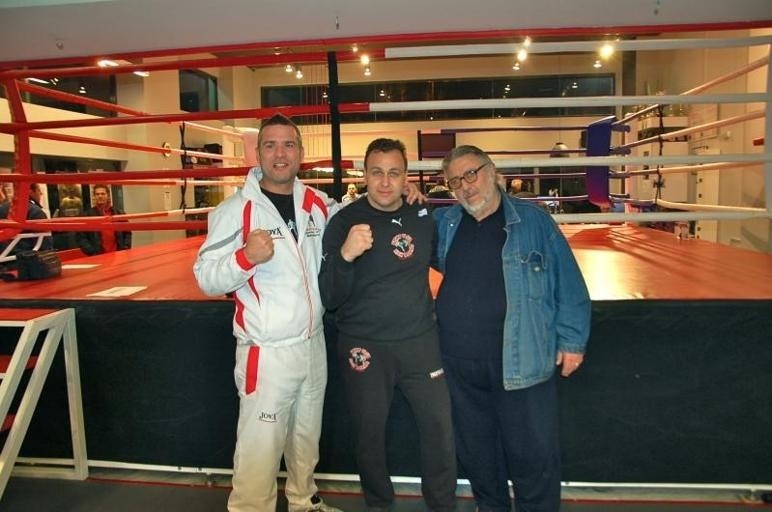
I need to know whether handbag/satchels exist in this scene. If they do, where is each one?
[16,249,60,280]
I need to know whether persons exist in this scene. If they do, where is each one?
[77,184,132,256]
[509,178,560,214]
[0,182,49,271]
[342,183,360,202]
[317,138,459,512]
[430,143,593,512]
[192,115,431,512]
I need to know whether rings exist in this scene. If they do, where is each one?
[575,362,580,366]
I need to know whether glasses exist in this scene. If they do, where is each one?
[447,162,488,189]
